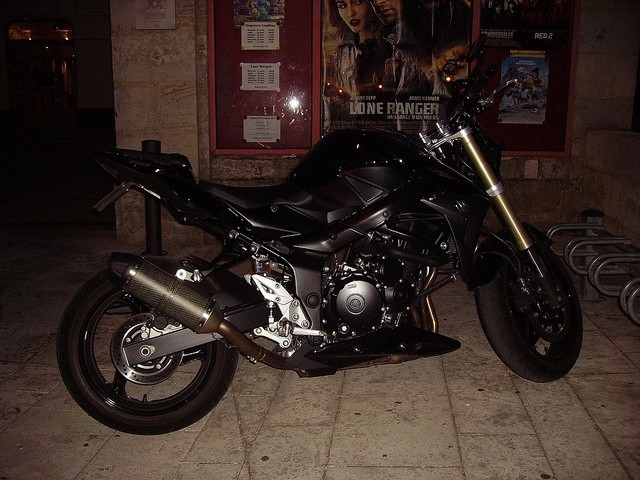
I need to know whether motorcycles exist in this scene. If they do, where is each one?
[55,34,583,436]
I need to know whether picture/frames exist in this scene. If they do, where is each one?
[319,0,473,142]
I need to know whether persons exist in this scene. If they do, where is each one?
[372,1,400,27]
[334,0,371,35]
[495,1,516,13]
